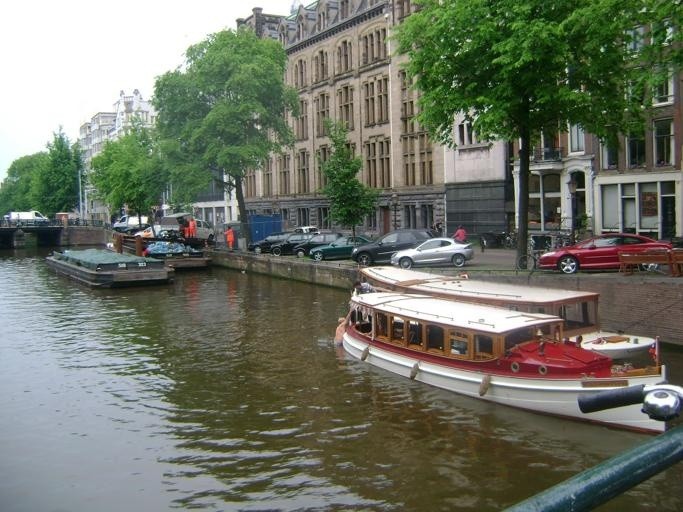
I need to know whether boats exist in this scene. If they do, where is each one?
[355,262,659,369]
[102,233,213,272]
[44,247,176,289]
[333,262,668,436]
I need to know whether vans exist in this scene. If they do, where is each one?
[291,226,318,236]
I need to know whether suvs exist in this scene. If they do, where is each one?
[291,232,344,259]
[270,231,320,257]
[350,227,442,265]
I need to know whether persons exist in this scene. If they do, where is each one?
[222,226,235,253]
[450,224,467,242]
[188,219,197,238]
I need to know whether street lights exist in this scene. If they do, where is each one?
[77,167,87,226]
[390,193,399,230]
[565,175,579,244]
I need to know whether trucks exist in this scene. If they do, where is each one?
[6,210,50,226]
[111,212,215,242]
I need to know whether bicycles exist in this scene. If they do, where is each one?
[515,238,556,271]
[576,383,683,421]
[498,230,534,249]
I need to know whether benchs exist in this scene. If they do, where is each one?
[616,246,683,277]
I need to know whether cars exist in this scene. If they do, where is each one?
[309,234,375,261]
[247,231,296,254]
[535,232,673,274]
[389,236,474,268]
